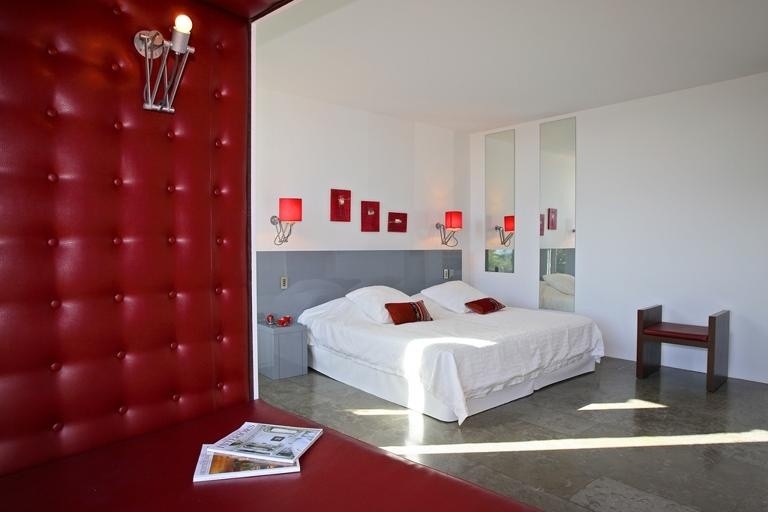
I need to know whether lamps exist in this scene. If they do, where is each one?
[494,215,514,246]
[270,198,302,245]
[133,13,196,114]
[435,210,462,246]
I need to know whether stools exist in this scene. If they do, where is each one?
[636,303,729,390]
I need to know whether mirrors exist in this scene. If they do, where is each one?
[539,116,575,312]
[484,128,516,273]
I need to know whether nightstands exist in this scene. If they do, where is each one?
[257,322,308,380]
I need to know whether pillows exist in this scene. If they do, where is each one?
[385,300,432,325]
[542,272,575,295]
[421,281,488,313]
[346,285,414,324]
[465,297,505,313]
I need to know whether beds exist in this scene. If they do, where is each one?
[298,294,605,423]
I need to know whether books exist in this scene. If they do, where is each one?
[192,444,301,482]
[207,421,324,464]
